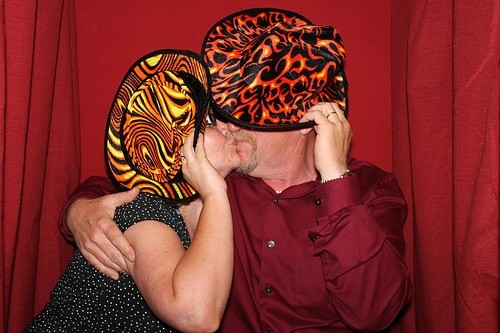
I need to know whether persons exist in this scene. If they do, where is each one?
[20,71,242,333]
[57,44,414,333]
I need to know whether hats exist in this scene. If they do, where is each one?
[199,6,349,132]
[105,46,210,206]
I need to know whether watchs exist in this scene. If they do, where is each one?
[320,169,356,187]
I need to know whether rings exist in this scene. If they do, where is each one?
[181,156,185,160]
[326,111,338,119]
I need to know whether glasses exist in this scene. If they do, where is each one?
[203,114,217,126]
